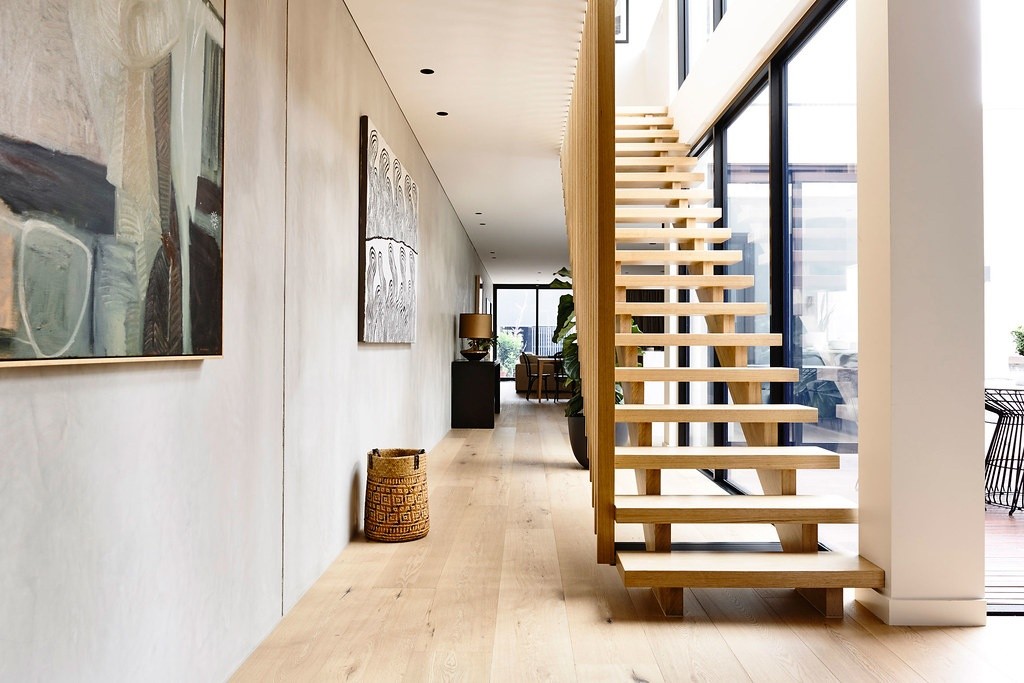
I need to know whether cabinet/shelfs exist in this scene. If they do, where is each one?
[451,359,500,429]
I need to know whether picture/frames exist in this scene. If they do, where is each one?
[614,0,629,43]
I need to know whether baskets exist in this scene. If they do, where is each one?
[363,448,431,543]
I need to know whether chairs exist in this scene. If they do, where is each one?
[554,351,576,403]
[522,352,550,401]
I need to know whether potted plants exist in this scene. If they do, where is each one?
[548,265,645,469]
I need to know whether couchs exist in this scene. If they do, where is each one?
[515,354,571,391]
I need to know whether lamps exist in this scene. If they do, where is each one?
[459,313,492,361]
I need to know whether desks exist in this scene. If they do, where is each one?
[537,358,569,402]
